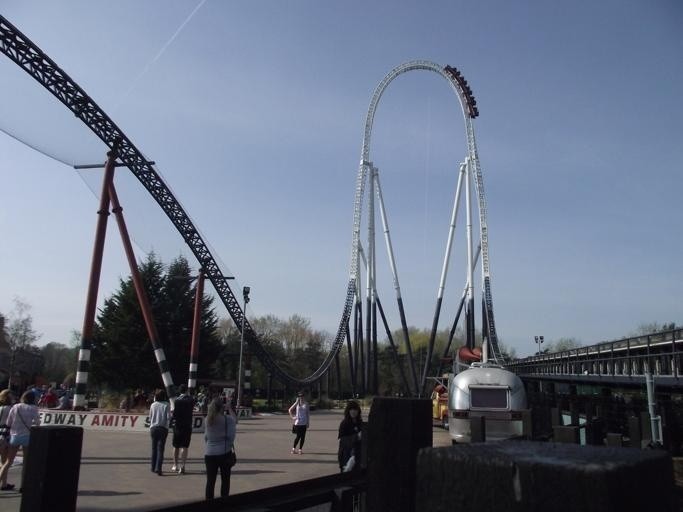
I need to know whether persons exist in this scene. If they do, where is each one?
[288,392,310,454]
[171,384,194,475]
[149,389,171,475]
[203,395,237,500]
[0,380,69,493]
[126,386,165,410]
[337,401,363,472]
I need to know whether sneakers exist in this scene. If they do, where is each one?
[291,448,302,455]
[171,463,185,474]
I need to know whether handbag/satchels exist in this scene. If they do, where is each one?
[292,424,297,433]
[225,452,236,468]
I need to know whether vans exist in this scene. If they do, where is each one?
[448,368,531,445]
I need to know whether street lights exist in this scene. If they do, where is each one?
[236,286,250,406]
[535,335,544,355]
